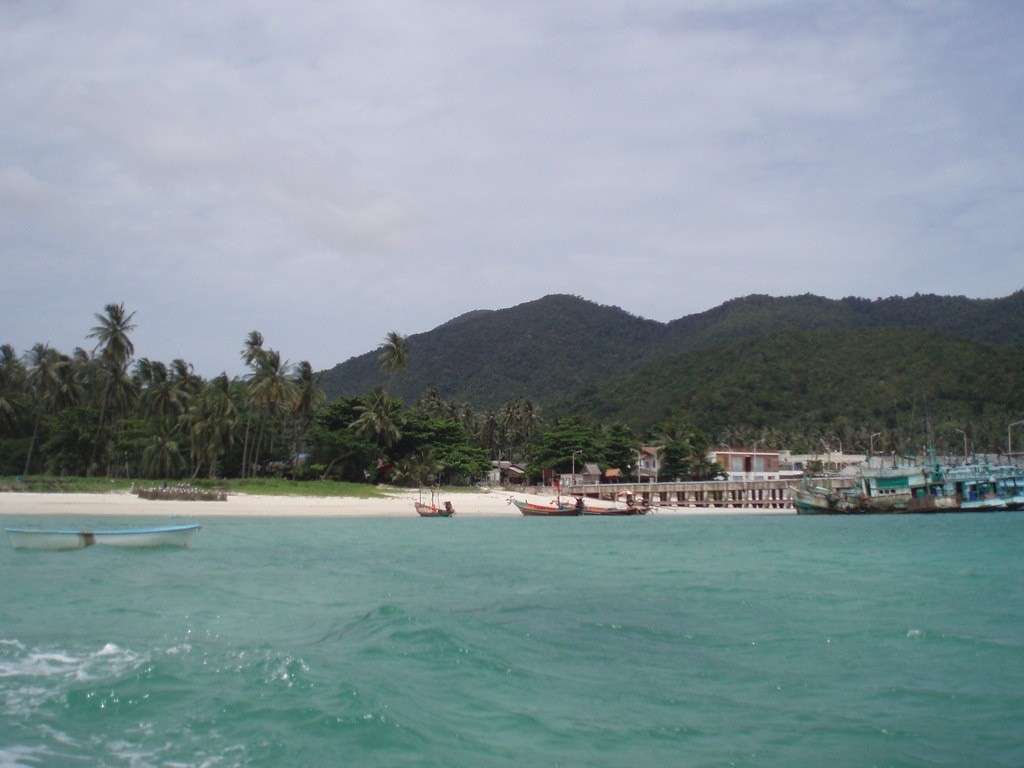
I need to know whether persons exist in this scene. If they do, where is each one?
[163,481,167,488]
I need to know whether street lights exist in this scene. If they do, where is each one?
[654,445,666,483]
[719,442,731,481]
[955,427,968,460]
[753,439,765,480]
[870,432,882,458]
[572,450,583,485]
[630,448,641,483]
[1007,420,1024,453]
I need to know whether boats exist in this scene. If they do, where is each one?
[414,500,456,518]
[4,523,204,550]
[789,442,1024,513]
[507,490,655,516]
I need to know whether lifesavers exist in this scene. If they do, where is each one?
[642,501,648,506]
[858,505,867,513]
[627,499,633,507]
[844,505,851,514]
